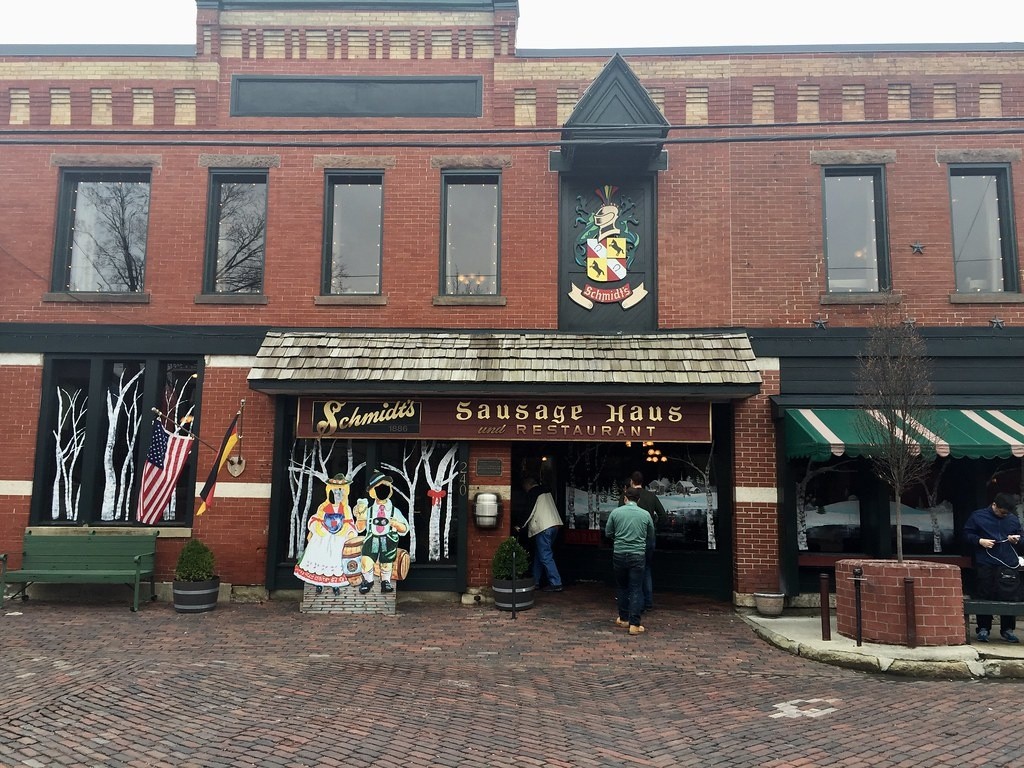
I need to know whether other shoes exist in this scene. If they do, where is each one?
[628,624,645,635]
[646,606,652,611]
[615,616,629,627]
[542,585,562,592]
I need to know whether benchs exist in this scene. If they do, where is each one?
[0,530,161,613]
[963,600,1024,645]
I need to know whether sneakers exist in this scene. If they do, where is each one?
[976,628,990,642]
[999,628,1021,644]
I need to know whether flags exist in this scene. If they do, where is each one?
[136,412,195,525]
[197,407,244,518]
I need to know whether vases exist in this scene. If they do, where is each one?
[753,592,784,616]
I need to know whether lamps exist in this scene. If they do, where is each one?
[472,492,503,531]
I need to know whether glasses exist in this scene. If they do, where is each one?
[997,508,1010,515]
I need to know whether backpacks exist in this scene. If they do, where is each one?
[991,565,1024,602]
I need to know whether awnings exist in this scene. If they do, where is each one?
[781,406,1024,464]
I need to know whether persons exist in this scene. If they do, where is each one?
[618,471,668,614]
[513,475,564,592]
[963,491,1024,644]
[603,488,655,635]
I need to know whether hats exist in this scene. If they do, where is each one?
[625,487,640,496]
[632,471,643,480]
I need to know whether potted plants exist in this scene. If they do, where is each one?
[492,536,536,611]
[832,282,967,648]
[171,538,220,613]
